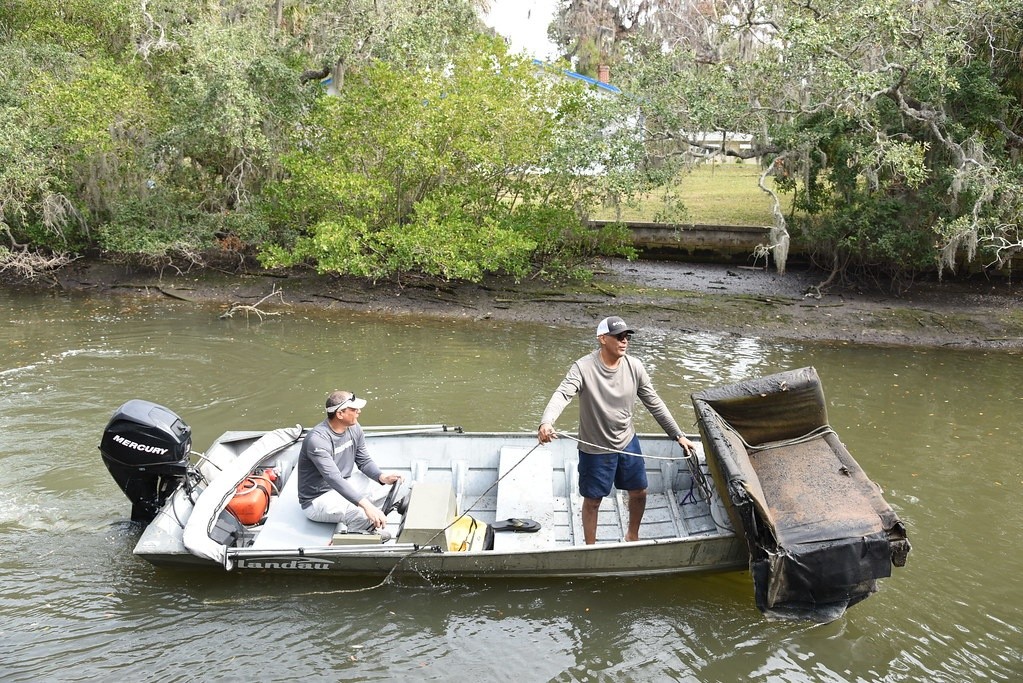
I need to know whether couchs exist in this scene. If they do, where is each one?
[690,365,911,626]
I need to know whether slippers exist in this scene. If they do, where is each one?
[490,518,541,532]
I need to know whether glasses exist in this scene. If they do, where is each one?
[604,333,631,341]
[335,391,356,413]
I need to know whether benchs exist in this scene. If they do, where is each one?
[252,461,336,547]
[493,445,555,549]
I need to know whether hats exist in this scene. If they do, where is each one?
[597,316,635,337]
[325,391,368,412]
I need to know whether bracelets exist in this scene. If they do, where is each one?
[675,432,686,441]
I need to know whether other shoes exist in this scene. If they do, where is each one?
[368,528,391,543]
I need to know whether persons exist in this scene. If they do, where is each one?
[537,316,696,545]
[298,391,405,543]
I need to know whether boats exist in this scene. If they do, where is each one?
[97,399,882,581]
